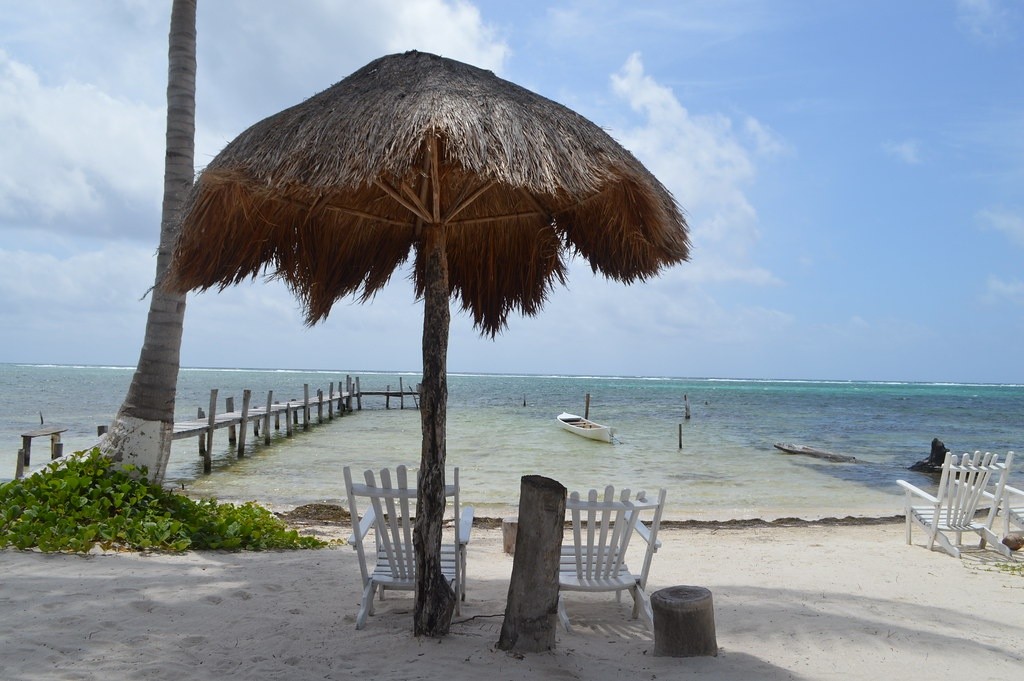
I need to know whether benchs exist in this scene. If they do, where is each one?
[568,422,586,425]
[575,424,592,429]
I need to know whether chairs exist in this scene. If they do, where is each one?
[558,485,666,633]
[342,465,474,631]
[895,451,1014,560]
[995,483,1024,538]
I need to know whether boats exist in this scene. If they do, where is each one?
[555,411,614,443]
[773,442,857,461]
